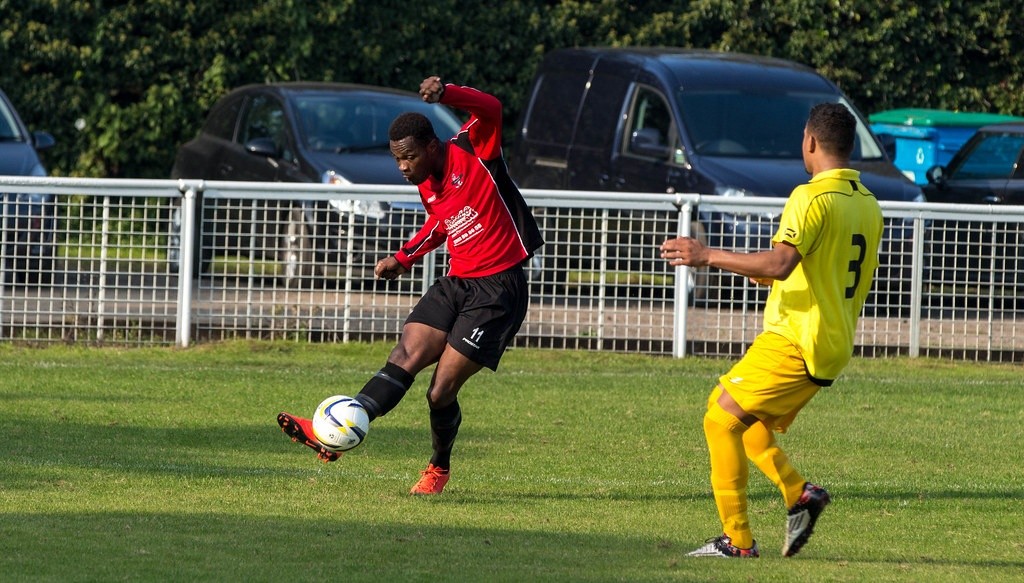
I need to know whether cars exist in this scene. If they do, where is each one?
[0,90,60,289]
[158,81,462,293]
[861,106,1024,311]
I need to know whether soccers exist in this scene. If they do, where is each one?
[311,394,370,453]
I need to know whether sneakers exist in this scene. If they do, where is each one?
[276,412,343,463]
[684,534,759,558]
[410,464,450,495]
[782,482,830,558]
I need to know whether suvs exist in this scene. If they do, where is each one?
[504,45,937,319]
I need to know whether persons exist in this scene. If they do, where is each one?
[659,103,884,558]
[277,74,545,495]
